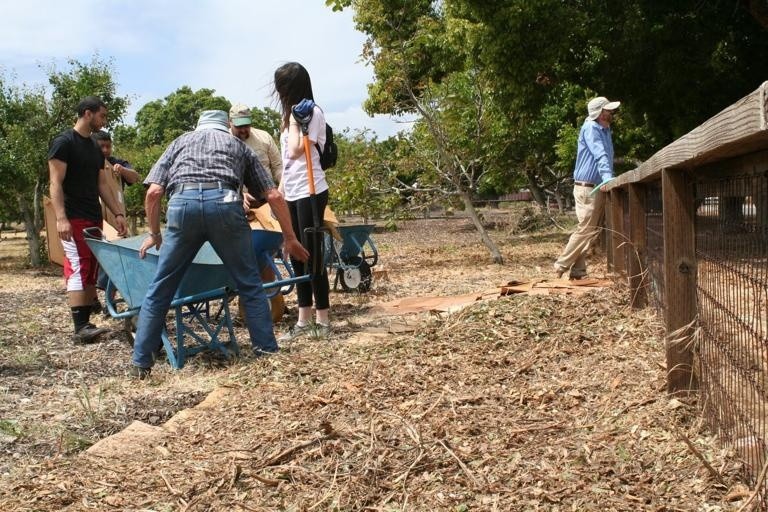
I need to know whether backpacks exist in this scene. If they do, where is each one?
[313,122,339,172]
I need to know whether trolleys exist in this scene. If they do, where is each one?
[272,221,380,296]
[79,223,315,370]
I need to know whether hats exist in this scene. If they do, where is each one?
[227,104,253,129]
[192,108,232,135]
[585,95,622,118]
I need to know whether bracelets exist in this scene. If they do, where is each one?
[149,231,161,237]
[115,214,124,219]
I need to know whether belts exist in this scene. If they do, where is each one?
[574,183,594,187]
[172,183,244,199]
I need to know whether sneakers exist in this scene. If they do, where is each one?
[73,322,108,344]
[129,365,151,381]
[276,323,314,342]
[306,322,330,341]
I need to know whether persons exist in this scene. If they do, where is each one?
[92,130,141,318]
[228,104,286,323]
[46,96,128,344]
[127,110,310,380]
[273,62,332,342]
[553,97,621,280]
